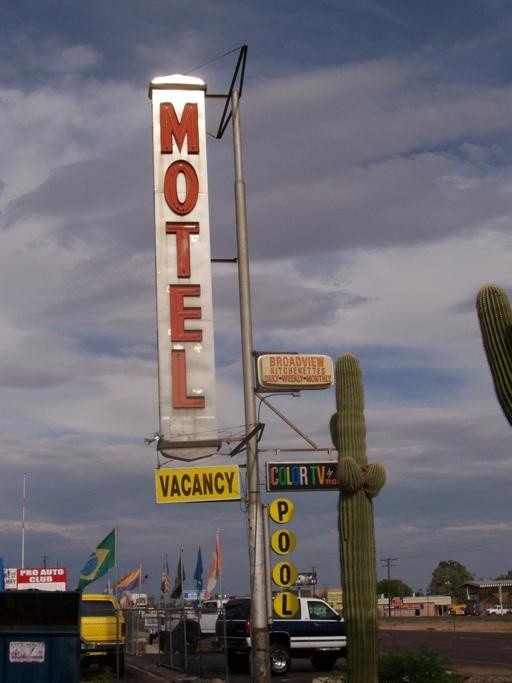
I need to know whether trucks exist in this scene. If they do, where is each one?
[139,597,230,656]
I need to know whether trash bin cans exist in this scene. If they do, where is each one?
[416,609,419,616]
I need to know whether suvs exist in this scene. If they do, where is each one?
[211,593,349,676]
[78,592,128,677]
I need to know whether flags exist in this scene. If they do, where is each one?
[78,529,222,609]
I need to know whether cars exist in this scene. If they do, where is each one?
[486,603,511,616]
